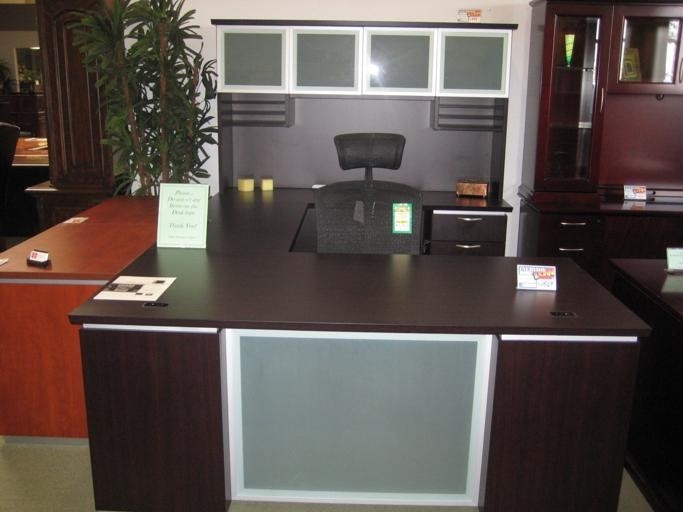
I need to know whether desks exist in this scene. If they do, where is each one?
[3,195,160,439]
[67,190,683,512]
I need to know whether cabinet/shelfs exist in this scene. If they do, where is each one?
[362,24,515,96]
[519,0,681,260]
[210,18,365,96]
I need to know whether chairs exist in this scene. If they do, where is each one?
[313,131,426,253]
